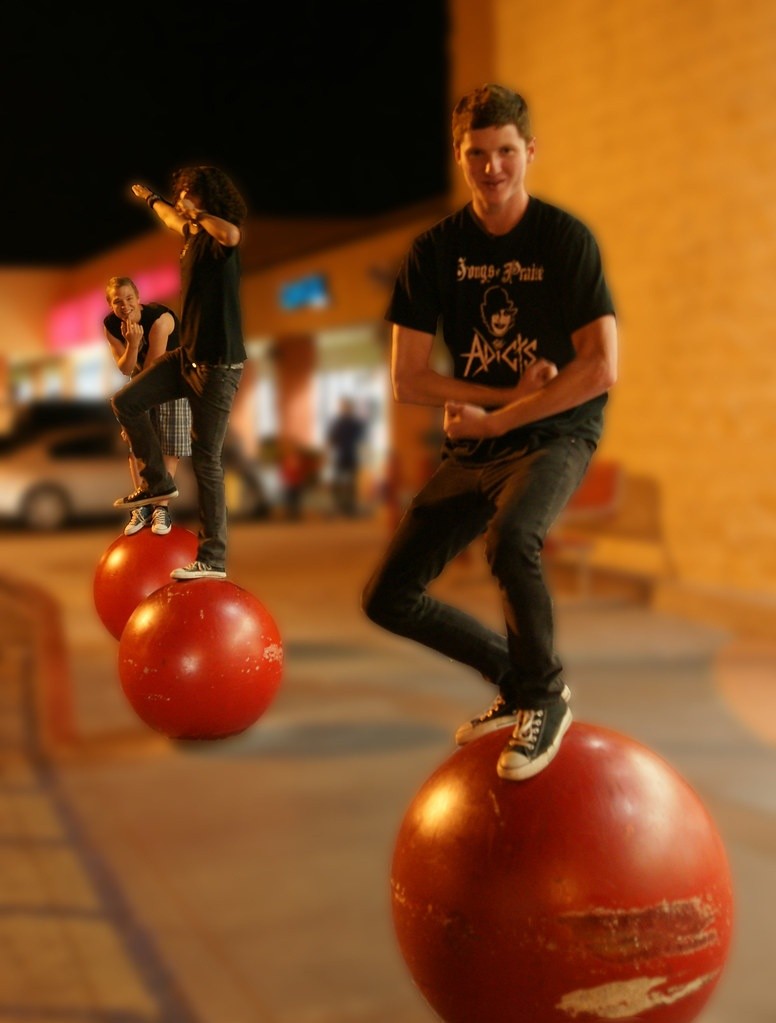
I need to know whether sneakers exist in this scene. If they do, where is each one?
[112,471,180,507]
[150,503,174,535]
[497,695,574,782]
[454,684,572,746]
[123,503,155,536]
[169,559,227,580]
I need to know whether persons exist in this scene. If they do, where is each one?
[256,436,322,519]
[130,160,247,580]
[360,82,621,784]
[101,273,196,537]
[326,398,367,515]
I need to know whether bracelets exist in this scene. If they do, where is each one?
[191,210,207,229]
[145,194,161,208]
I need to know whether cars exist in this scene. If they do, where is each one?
[0,418,258,537]
